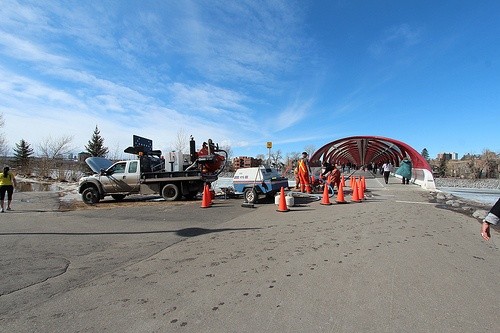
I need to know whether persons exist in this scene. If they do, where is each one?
[395,157,412,184]
[481,197,500,240]
[343,161,384,175]
[296,152,313,194]
[294,167,301,189]
[321,162,341,193]
[0,166,17,212]
[381,159,392,184]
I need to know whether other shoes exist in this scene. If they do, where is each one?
[1,209,4,213]
[7,207,10,210]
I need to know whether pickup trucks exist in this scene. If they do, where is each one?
[77,136,228,206]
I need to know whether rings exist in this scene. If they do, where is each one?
[481,233,483,236]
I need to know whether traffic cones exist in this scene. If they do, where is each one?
[319,183,333,205]
[199,183,212,208]
[341,175,346,187]
[335,182,348,204]
[276,187,291,212]
[351,182,362,203]
[357,182,367,200]
[350,175,369,194]
